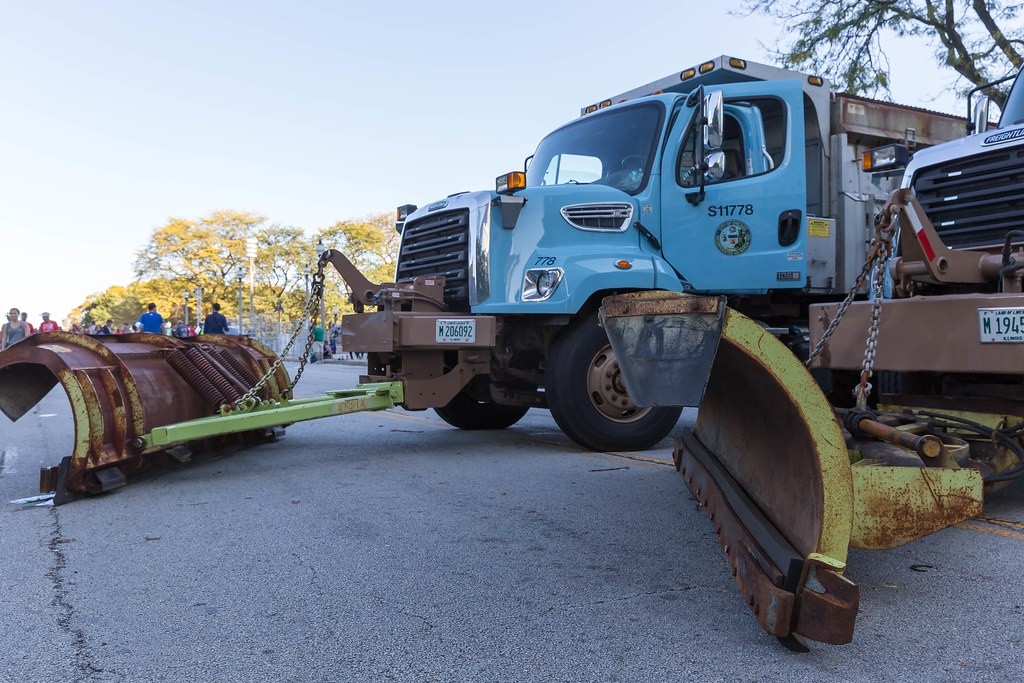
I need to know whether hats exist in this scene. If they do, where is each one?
[5,311,10,317]
[41,313,50,316]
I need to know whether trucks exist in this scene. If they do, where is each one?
[785,53,1024,511]
[386,51,996,450]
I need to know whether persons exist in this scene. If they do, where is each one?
[313,322,368,364]
[0,307,35,351]
[71,320,204,335]
[204,303,229,334]
[38,312,58,333]
[138,303,165,335]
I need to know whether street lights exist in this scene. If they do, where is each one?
[236,266,244,335]
[315,239,327,362]
[183,289,190,325]
[332,304,339,324]
